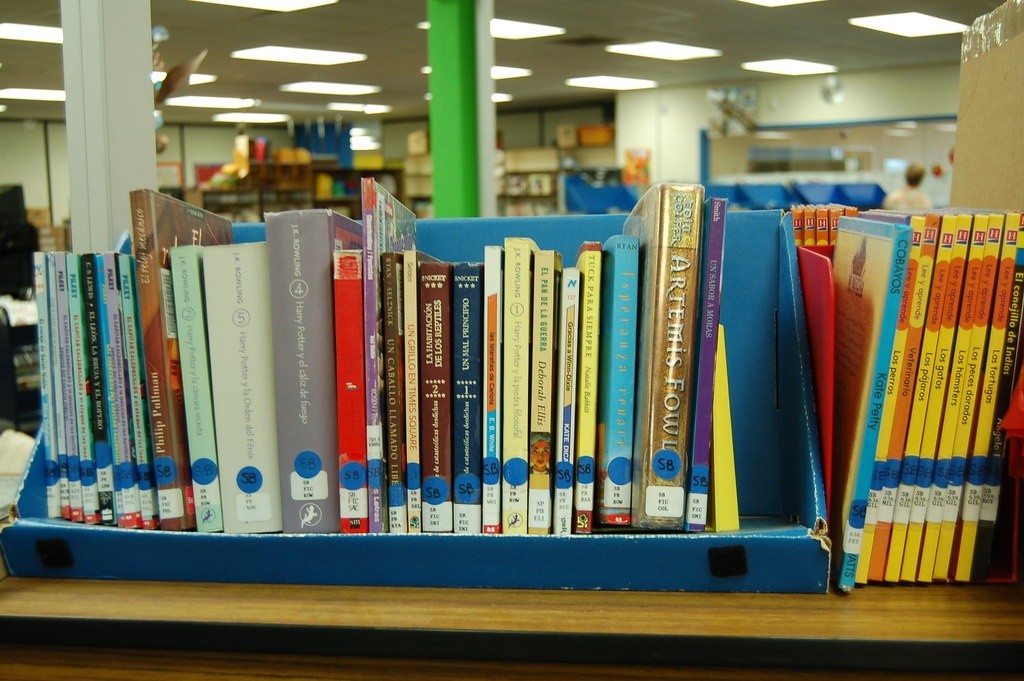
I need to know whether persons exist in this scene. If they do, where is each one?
[0,187,41,417]
[883,163,934,210]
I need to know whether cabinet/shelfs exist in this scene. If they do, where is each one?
[497,147,624,215]
[184,165,403,224]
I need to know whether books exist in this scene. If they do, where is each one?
[33,170,1024,591]
